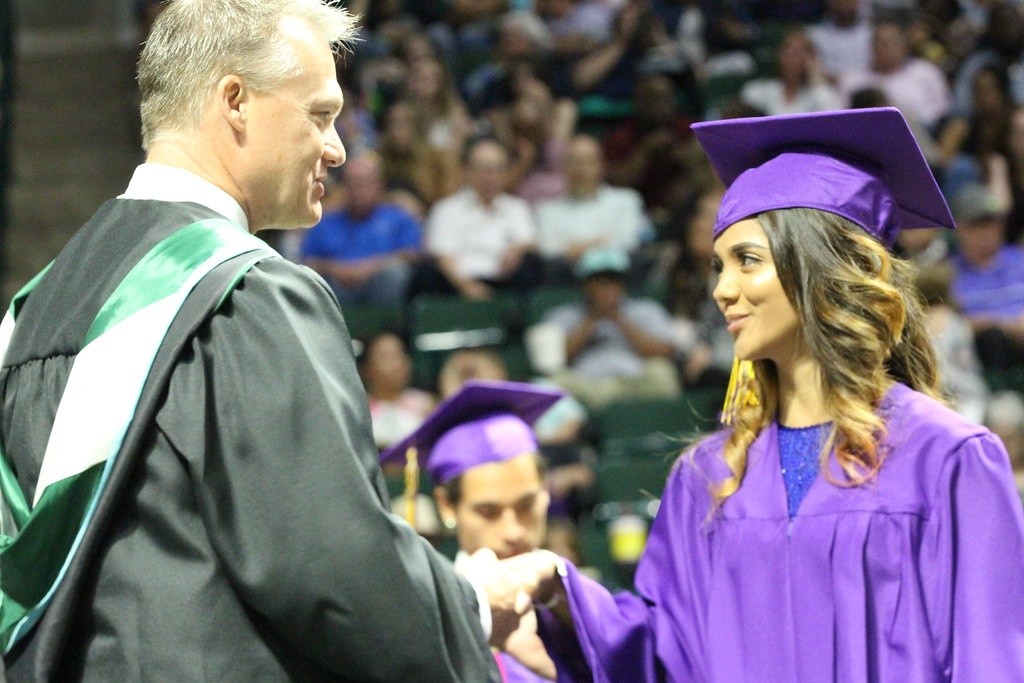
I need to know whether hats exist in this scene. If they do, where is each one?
[377,378,566,538]
[689,107,955,428]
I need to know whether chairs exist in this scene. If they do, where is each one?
[328,287,733,602]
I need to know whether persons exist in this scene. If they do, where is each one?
[941,185,1024,370]
[299,148,421,308]
[357,329,437,453]
[0,0,560,683]
[738,28,841,116]
[421,133,537,304]
[542,244,681,380]
[843,21,951,133]
[244,0,1024,567]
[377,379,569,683]
[534,132,656,284]
[498,107,1024,683]
